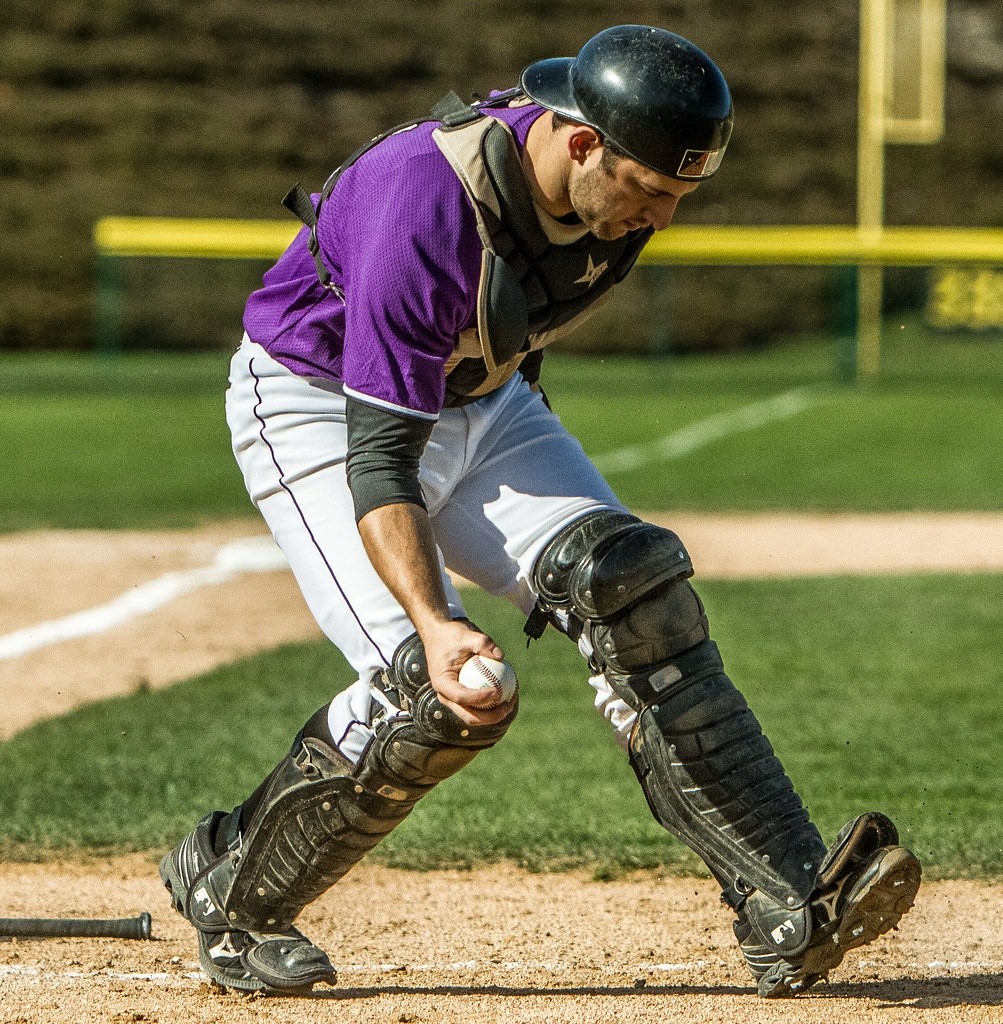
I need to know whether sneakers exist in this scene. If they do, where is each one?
[734,846,922,998]
[158,810,339,998]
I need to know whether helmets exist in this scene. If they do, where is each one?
[519,24,734,182]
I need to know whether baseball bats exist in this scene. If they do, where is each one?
[0,912,153,940]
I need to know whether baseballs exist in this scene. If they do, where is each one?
[458,654,518,711]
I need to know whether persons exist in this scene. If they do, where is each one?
[155,21,924,1001]
